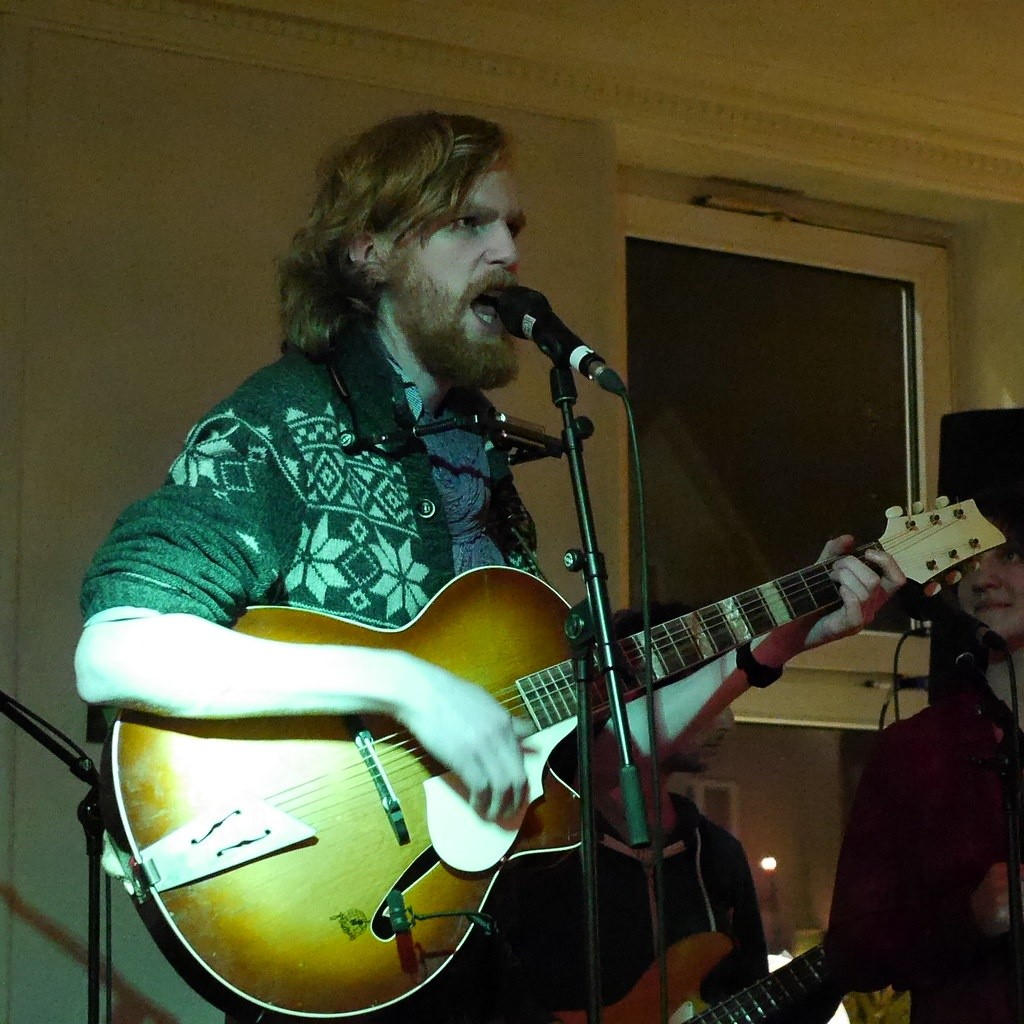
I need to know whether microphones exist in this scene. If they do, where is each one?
[898,586,1007,655]
[495,284,627,399]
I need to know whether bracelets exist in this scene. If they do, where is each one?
[735,638,783,690]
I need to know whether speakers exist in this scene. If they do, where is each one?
[925,409,1024,705]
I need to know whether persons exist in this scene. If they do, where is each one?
[825,480,1024,1024]
[512,608,769,1024]
[74,110,906,1024]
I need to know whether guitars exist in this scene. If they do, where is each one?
[553,930,834,1022]
[98,489,1013,1022]
